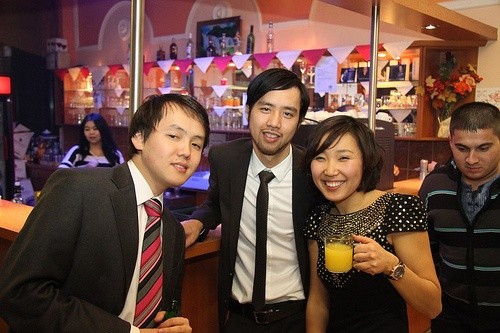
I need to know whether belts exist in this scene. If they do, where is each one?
[231,301,306,323]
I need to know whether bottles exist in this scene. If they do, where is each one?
[11,181,24,203]
[125,19,276,64]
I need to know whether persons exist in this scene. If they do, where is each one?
[177,68,337,333]
[56,113,126,172]
[0,92,212,333]
[415,101,500,333]
[300,115,444,333]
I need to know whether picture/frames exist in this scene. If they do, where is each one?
[194,14,243,57]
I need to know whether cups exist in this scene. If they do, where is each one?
[25,74,127,164]
[188,87,246,129]
[298,62,417,132]
[324,234,359,274]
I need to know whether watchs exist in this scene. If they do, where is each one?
[386,259,405,281]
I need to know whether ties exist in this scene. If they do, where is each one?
[133,198,163,330]
[252,171,274,311]
